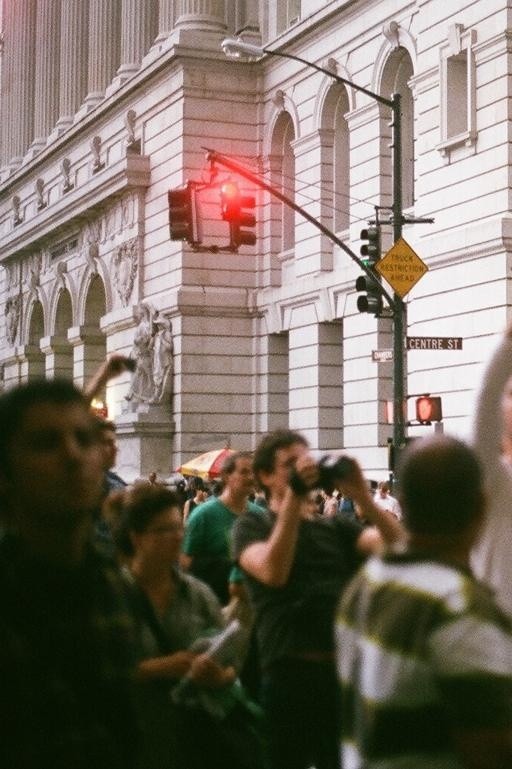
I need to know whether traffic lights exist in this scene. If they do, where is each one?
[219,176,243,224]
[416,396,443,422]
[356,226,383,314]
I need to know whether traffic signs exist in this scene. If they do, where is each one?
[371,336,463,363]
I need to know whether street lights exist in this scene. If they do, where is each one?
[219,36,408,460]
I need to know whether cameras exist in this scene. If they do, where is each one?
[318,457,352,486]
